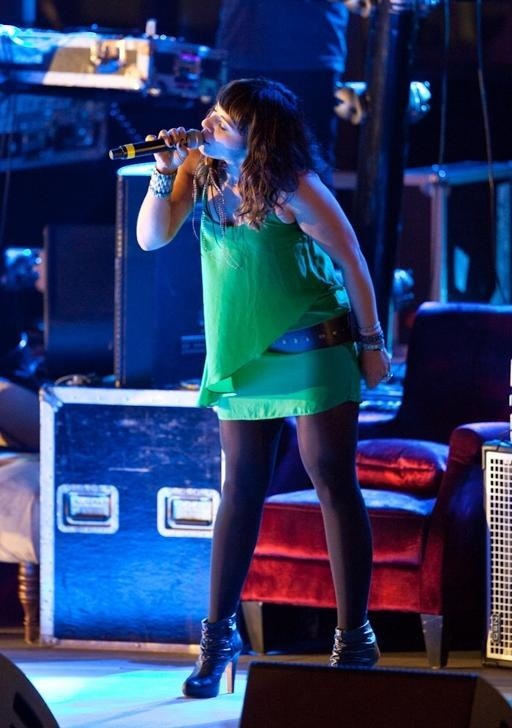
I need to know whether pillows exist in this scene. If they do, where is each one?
[356,439,449,493]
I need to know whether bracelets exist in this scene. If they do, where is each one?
[357,322,385,352]
[148,168,179,200]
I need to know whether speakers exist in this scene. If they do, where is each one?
[0,651,59,728]
[115,161,204,386]
[43,223,117,378]
[238,659,512,728]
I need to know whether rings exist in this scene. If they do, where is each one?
[379,372,393,384]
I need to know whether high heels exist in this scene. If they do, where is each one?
[183,613,244,697]
[328,620,381,666]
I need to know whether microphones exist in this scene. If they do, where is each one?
[109,128,203,160]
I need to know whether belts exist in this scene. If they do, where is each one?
[268,312,355,352]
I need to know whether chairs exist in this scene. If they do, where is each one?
[239,303,511,668]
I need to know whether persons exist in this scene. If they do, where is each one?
[137,81,394,697]
[210,0,375,170]
